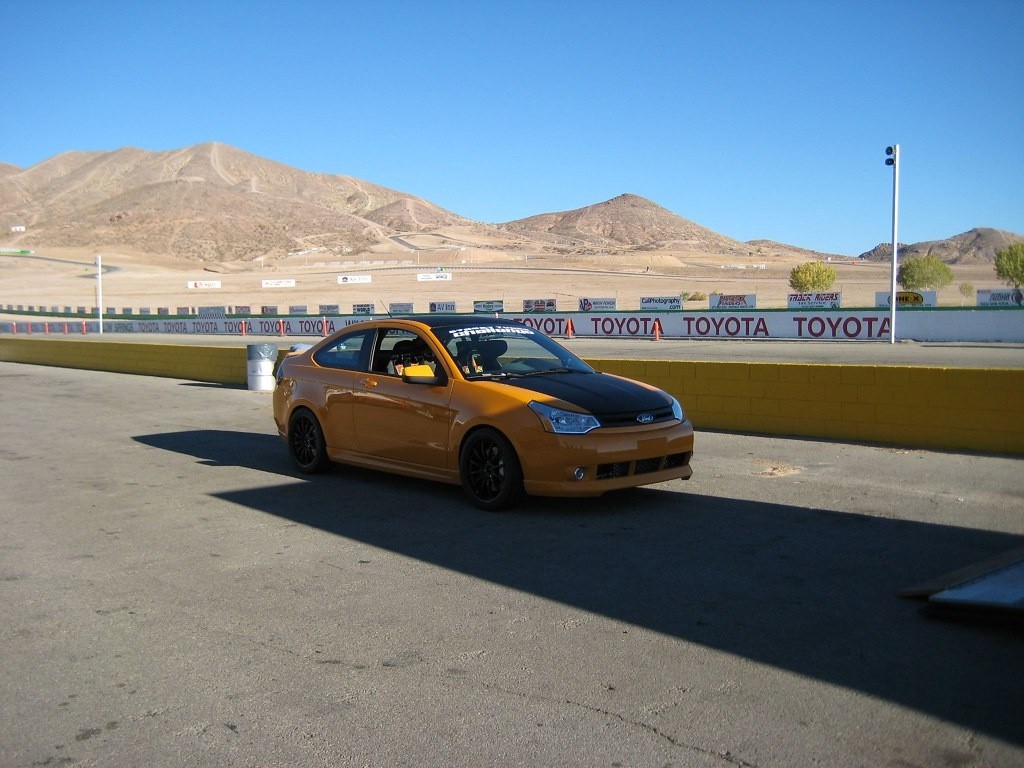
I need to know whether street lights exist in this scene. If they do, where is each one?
[885,144,900,345]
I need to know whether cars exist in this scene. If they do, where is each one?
[274,312,696,509]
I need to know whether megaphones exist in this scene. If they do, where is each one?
[886,146,896,155]
[885,158,895,166]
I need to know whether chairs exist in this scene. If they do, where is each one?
[391,340,435,378]
[457,339,508,373]
